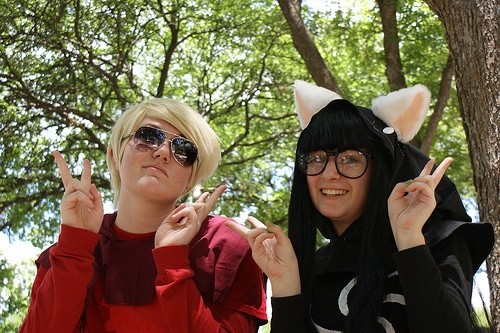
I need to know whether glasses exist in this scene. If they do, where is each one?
[122,126,200,168]
[296,147,376,179]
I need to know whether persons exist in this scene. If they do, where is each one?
[18,98,270,333]
[270,79,497,333]
